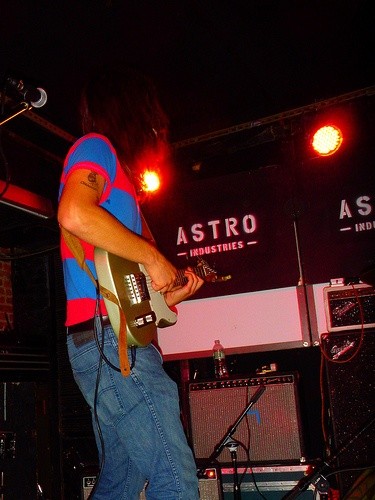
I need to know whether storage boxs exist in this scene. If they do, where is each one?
[221,464,320,500]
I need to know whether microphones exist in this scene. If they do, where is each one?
[7,78,47,108]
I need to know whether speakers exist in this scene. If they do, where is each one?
[82,466,223,500]
[322,329,375,500]
[185,371,305,466]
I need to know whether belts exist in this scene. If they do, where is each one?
[68,316,111,335]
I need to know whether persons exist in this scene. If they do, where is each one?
[57,77,205,500]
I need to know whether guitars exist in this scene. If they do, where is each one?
[93,250,231,349]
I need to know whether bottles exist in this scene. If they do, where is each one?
[212,341,230,380]
